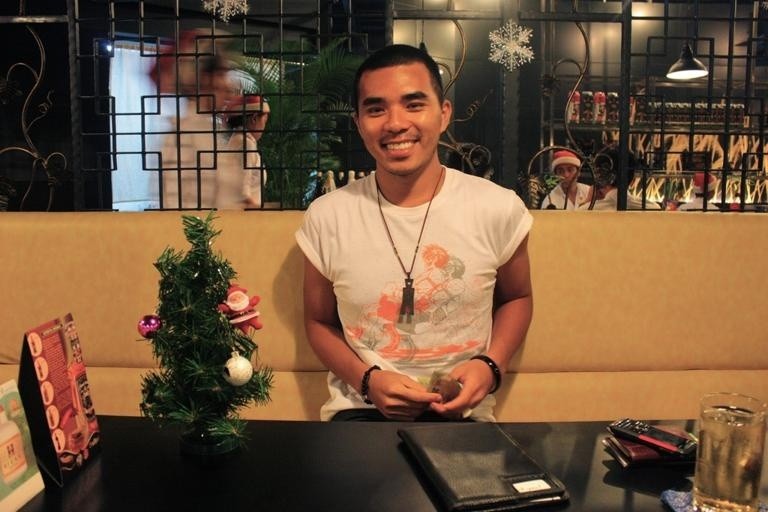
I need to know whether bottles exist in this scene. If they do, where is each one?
[68,322,82,364]
[30,335,37,354]
[49,410,55,426]
[37,361,44,377]
[42,385,50,402]
[313,169,376,202]
[55,432,61,450]
[566,89,620,124]
[0,405,27,486]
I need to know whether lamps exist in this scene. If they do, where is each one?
[662,2,710,83]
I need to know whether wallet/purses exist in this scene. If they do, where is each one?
[602,425,696,469]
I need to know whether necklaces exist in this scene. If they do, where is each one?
[374,166,443,317]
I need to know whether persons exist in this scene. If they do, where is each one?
[675,174,718,210]
[160,48,243,207]
[223,93,270,209]
[292,43,532,423]
[576,144,659,210]
[539,151,591,210]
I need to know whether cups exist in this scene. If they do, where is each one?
[694,390,768,511]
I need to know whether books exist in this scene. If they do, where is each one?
[395,420,569,512]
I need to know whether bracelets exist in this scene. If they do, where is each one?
[359,364,381,404]
[469,354,501,394]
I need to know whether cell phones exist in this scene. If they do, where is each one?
[607,416,697,457]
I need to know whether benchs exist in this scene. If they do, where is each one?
[0,204,768,425]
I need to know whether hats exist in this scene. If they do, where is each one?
[552,151,581,172]
[693,172,716,193]
[225,95,270,116]
